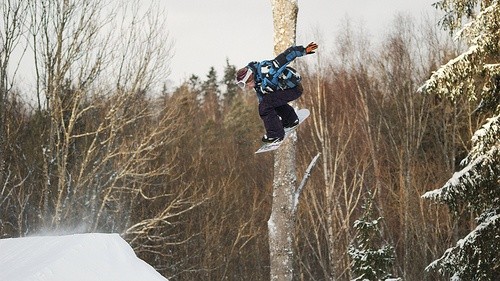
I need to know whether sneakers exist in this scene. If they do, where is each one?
[262,134,284,143]
[280,118,299,128]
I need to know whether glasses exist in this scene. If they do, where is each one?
[236,81,245,90]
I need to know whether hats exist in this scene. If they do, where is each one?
[236,68,254,85]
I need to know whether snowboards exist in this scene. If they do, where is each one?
[254,108,310,154]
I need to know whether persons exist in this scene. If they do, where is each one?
[237,42,318,143]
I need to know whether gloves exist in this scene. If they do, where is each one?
[305,42,318,55]
